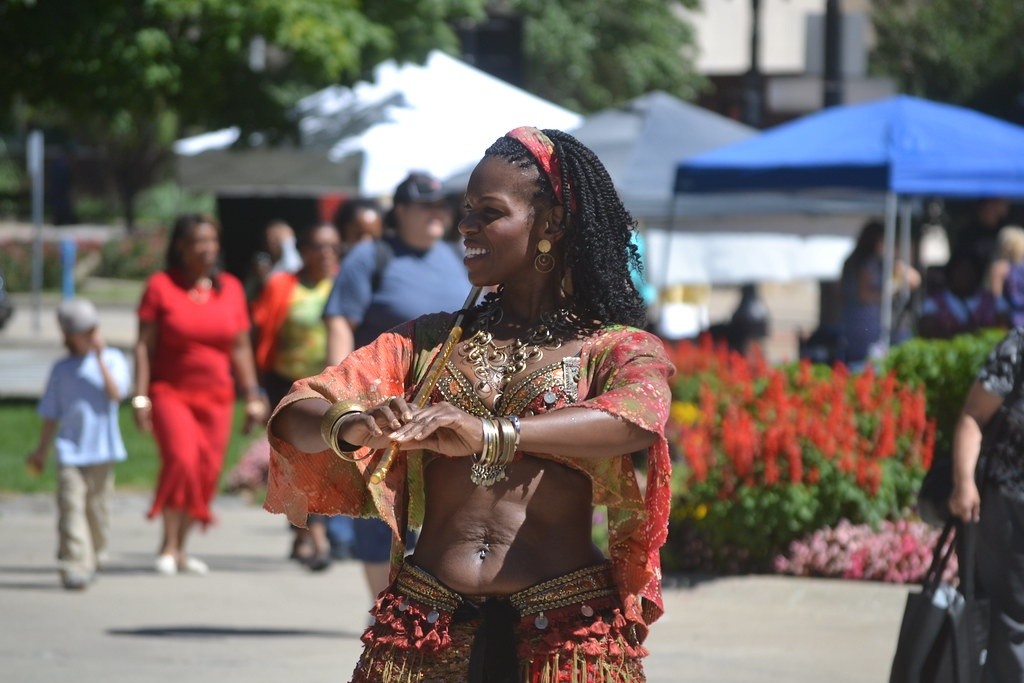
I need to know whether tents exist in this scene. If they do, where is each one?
[174,45,918,345]
[658,95,1024,358]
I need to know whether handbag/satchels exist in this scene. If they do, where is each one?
[887,513,996,683]
[919,437,988,529]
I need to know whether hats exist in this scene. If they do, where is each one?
[394,170,455,213]
[59,297,101,334]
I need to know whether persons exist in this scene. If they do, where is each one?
[955,202,1008,332]
[28,297,130,589]
[839,221,919,359]
[322,173,474,599]
[948,326,1024,683]
[252,222,342,571]
[989,227,1023,332]
[133,215,267,576]
[265,126,677,682]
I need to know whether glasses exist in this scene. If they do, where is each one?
[307,242,346,254]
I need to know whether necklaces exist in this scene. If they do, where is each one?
[458,291,587,414]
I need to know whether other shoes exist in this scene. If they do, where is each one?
[61,568,93,589]
[182,558,208,574]
[152,552,177,576]
[287,538,334,572]
[332,541,357,562]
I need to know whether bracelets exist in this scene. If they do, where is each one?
[470,416,521,486]
[132,396,152,410]
[320,401,376,463]
